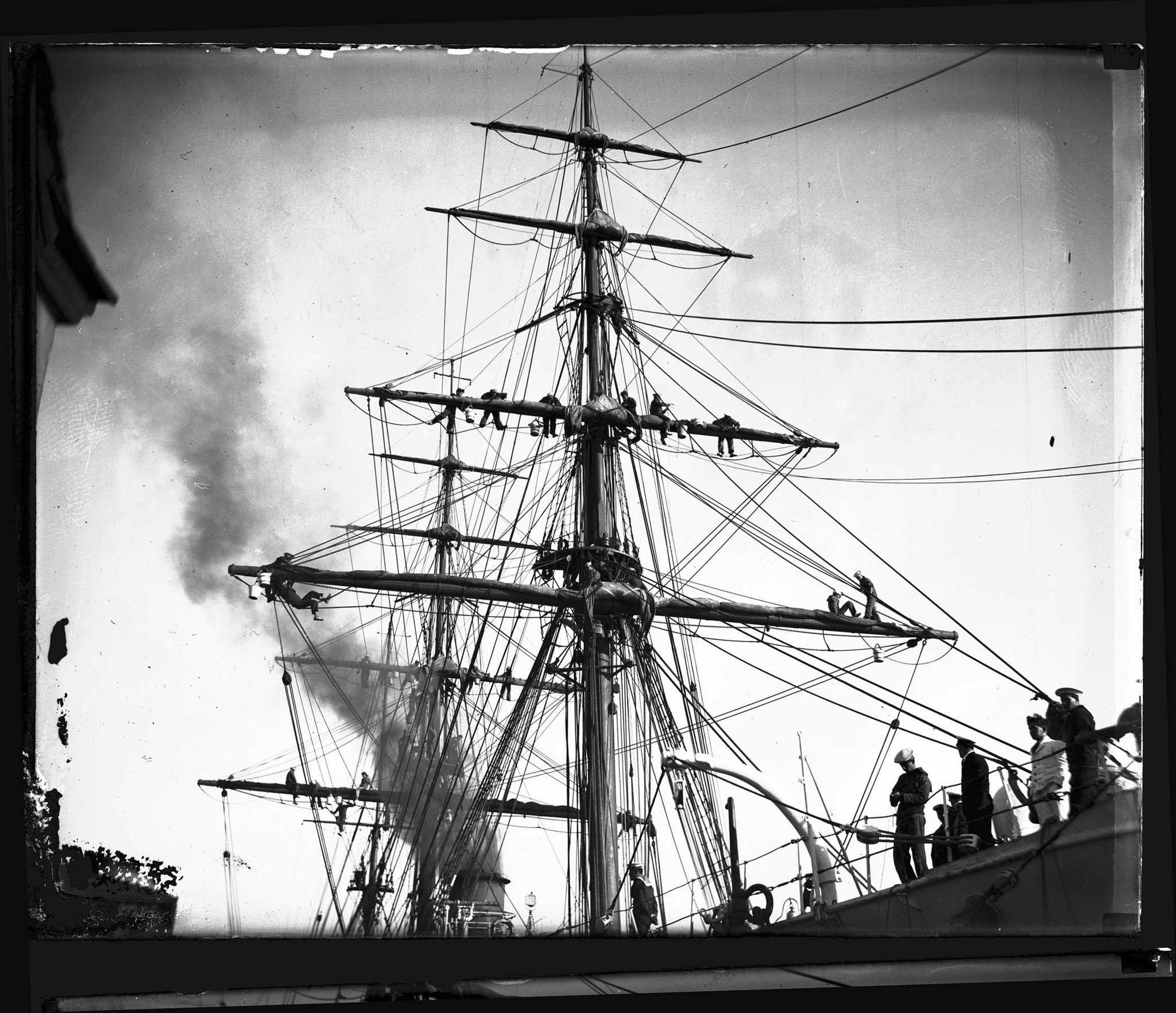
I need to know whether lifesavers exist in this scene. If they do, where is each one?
[745,883,774,925]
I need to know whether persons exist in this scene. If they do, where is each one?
[621,391,643,439]
[956,735,996,851]
[428,388,465,434]
[264,553,294,603]
[539,533,648,590]
[931,803,953,868]
[1047,687,1100,818]
[353,772,374,803]
[275,580,331,621]
[360,654,514,766]
[889,748,932,884]
[285,766,300,805]
[826,589,861,618]
[480,389,508,431]
[650,394,673,446]
[1026,715,1065,829]
[626,862,659,936]
[330,800,353,836]
[538,392,562,439]
[712,414,740,458]
[854,571,881,620]
[946,791,970,860]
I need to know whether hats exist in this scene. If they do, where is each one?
[1026,716,1050,727]
[894,749,913,764]
[955,735,975,748]
[626,862,644,870]
[933,804,950,811]
[946,792,962,801]
[1055,687,1083,699]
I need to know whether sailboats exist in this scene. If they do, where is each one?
[191,50,1148,927]
